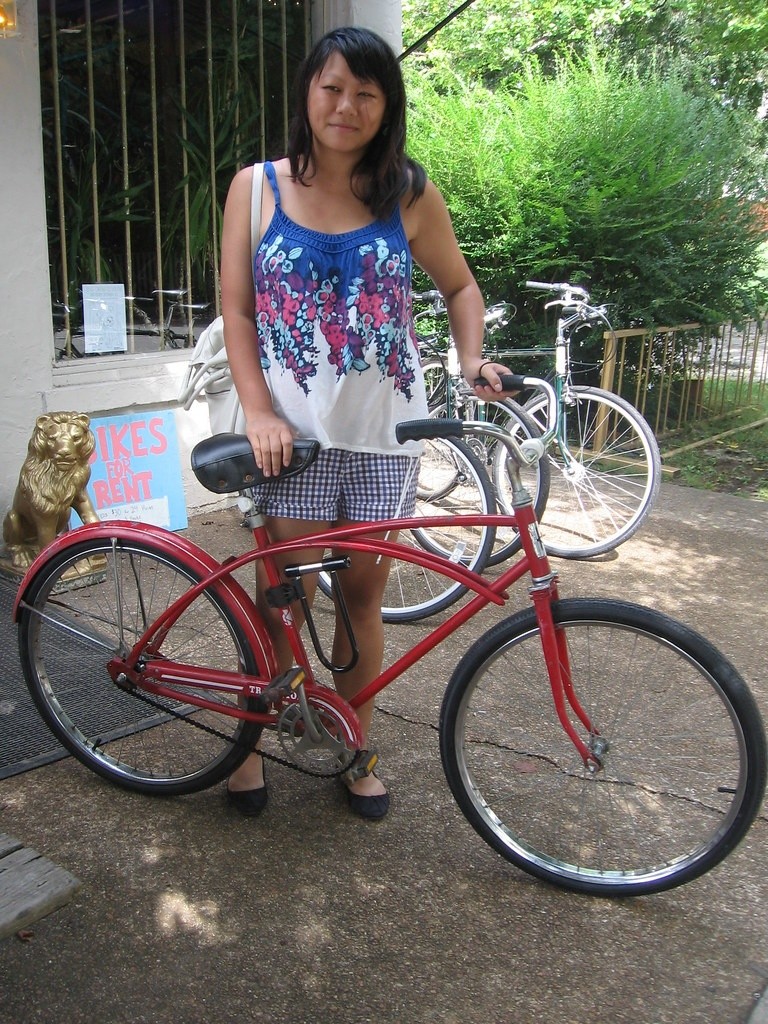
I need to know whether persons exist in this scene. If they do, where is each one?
[220,26,519,820]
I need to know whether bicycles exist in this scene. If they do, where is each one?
[10,375,768,900]
[315,282,662,623]
[53,288,215,361]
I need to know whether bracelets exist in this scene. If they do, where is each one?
[479,362,499,377]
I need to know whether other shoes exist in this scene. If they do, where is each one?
[336,753,389,820]
[226,753,268,817]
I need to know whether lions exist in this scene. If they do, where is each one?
[3,410,103,569]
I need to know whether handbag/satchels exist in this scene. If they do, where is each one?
[180,163,264,436]
